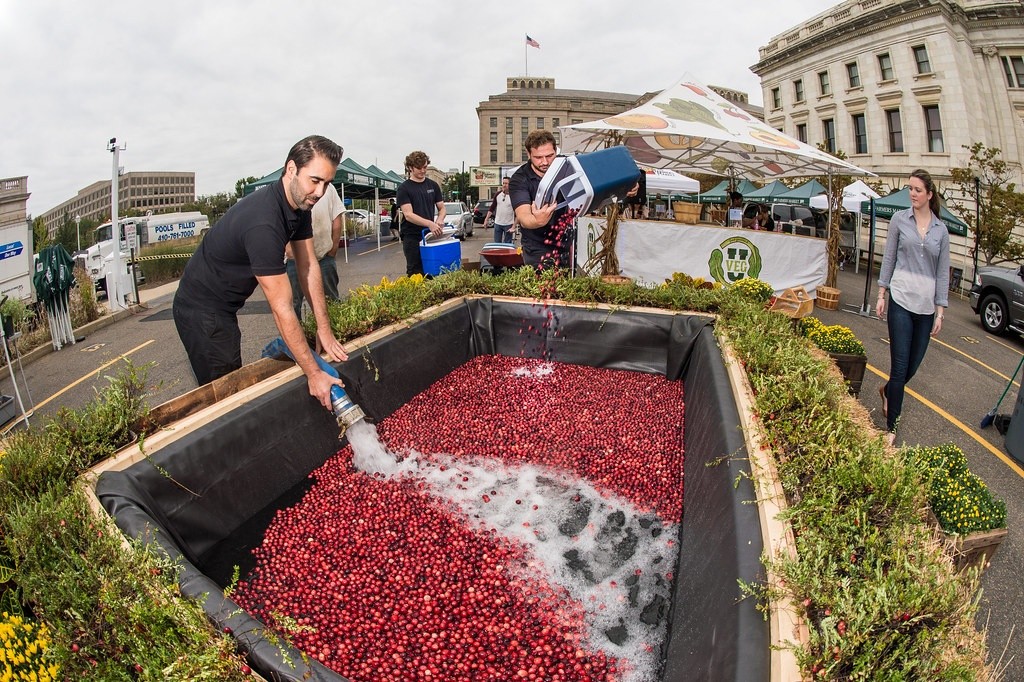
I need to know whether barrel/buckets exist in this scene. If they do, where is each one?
[672,202,703,224]
[816,286,842,310]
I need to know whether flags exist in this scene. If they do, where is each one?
[526,35,540,48]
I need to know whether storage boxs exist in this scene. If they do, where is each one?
[479,243,516,278]
[338,236,349,248]
[534,144,641,218]
[419,225,461,276]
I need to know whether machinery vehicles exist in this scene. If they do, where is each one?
[72,211,210,290]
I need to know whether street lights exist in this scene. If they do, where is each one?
[972,166,981,286]
[76,215,80,250]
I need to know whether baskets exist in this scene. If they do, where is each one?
[705,209,727,224]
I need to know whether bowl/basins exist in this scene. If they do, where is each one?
[478,249,523,267]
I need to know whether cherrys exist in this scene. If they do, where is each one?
[518,204,583,359]
[230,353,684,682]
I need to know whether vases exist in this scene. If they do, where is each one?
[899,449,1008,576]
[0,395,16,428]
[824,350,868,399]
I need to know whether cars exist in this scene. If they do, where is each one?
[471,200,496,228]
[433,202,473,241]
[970,265,1024,341]
[345,209,392,228]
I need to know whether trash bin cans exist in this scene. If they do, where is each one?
[1005,366,1024,462]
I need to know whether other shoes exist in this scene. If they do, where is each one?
[884,430,896,446]
[879,386,888,417]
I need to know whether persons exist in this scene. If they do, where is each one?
[726,192,745,229]
[650,193,666,219]
[285,182,347,329]
[623,200,647,219]
[874,170,950,447]
[759,206,775,231]
[484,177,518,245]
[508,129,575,280]
[396,152,446,281]
[172,135,349,411]
[389,198,400,242]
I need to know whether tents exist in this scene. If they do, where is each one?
[557,73,880,240]
[620,167,702,194]
[689,178,969,238]
[240,153,417,252]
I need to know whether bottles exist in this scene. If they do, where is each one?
[792,225,796,235]
[777,220,781,232]
[755,216,759,229]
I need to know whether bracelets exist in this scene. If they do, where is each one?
[935,314,944,321]
[877,291,885,295]
[877,297,885,299]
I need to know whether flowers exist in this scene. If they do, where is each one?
[898,440,1006,533]
[729,276,774,301]
[798,315,868,354]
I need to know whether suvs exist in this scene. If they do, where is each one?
[743,201,857,250]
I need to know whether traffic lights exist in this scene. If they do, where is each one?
[453,193,457,202]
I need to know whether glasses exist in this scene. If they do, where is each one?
[503,194,506,201]
